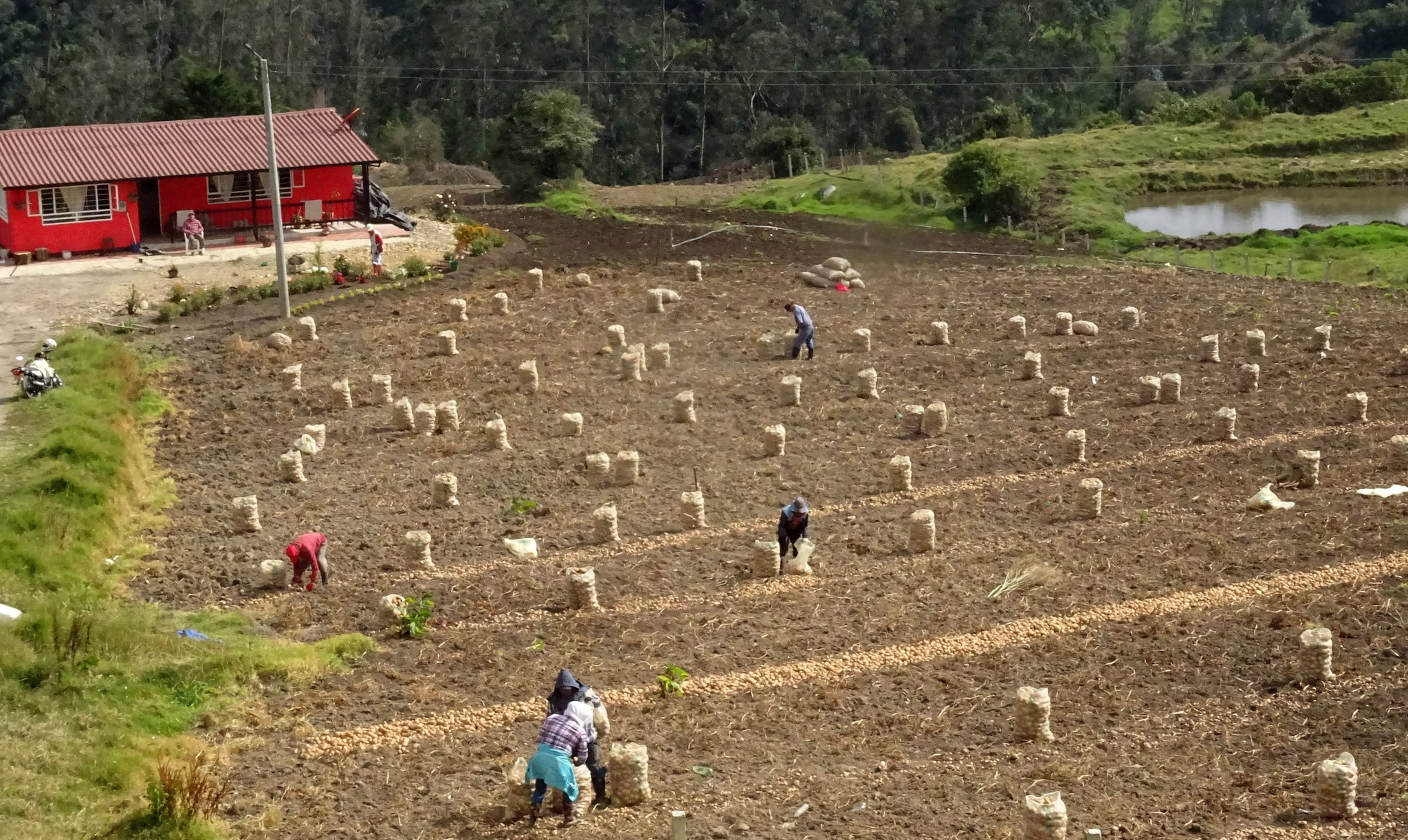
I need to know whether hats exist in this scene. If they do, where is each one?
[364,224,373,232]
[187,211,195,217]
[786,301,793,312]
[791,496,807,513]
[286,545,298,563]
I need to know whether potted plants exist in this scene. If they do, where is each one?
[127,182,137,203]
[258,234,272,247]
[168,264,178,278]
[10,190,25,211]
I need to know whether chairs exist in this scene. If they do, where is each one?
[184,232,204,252]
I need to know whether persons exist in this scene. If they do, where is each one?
[183,211,206,256]
[778,496,810,576]
[524,700,594,829]
[284,529,332,591]
[784,301,817,361]
[543,668,612,809]
[364,222,386,277]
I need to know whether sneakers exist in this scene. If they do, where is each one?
[780,559,785,571]
[564,817,577,826]
[531,810,542,819]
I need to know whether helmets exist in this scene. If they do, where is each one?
[41,338,57,352]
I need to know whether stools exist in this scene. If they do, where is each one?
[101,237,116,256]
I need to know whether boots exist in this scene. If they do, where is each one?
[371,264,383,278]
[806,347,815,360]
[789,345,801,360]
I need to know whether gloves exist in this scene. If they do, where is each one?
[307,583,314,591]
[785,537,790,545]
[794,328,800,333]
[291,577,302,583]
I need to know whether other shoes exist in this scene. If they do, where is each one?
[198,250,206,256]
[596,794,610,804]
[190,252,196,256]
